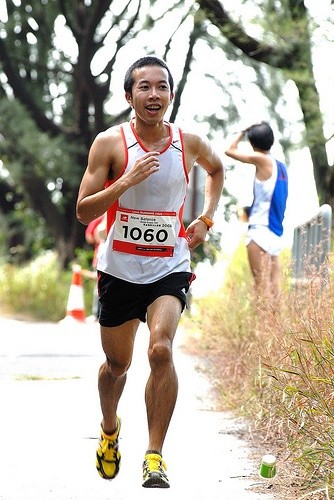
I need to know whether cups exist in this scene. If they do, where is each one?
[259,454,277,480]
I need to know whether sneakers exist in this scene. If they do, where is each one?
[142,451,170,488]
[95,417,121,479]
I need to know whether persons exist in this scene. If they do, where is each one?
[85,215,104,323]
[224,120,288,311]
[76,57,223,488]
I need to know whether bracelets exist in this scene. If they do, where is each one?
[198,214,214,231]
